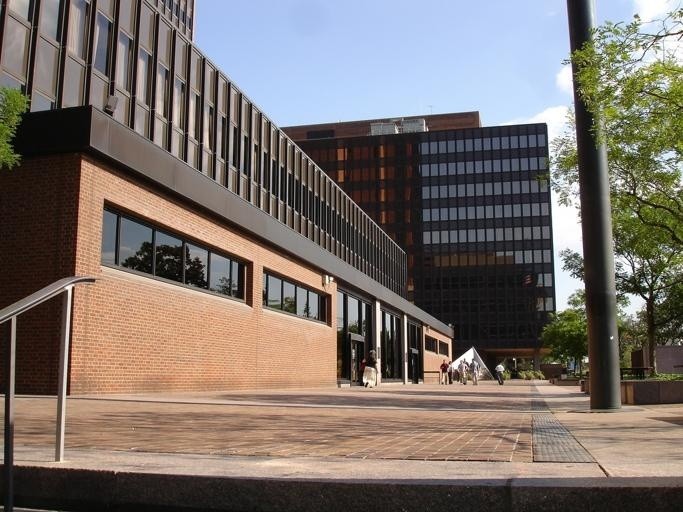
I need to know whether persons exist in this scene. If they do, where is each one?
[361,349,379,389]
[495,362,504,384]
[440,358,480,386]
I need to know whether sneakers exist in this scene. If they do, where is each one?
[365,382,372,388]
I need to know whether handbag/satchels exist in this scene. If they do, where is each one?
[360,362,365,368]
